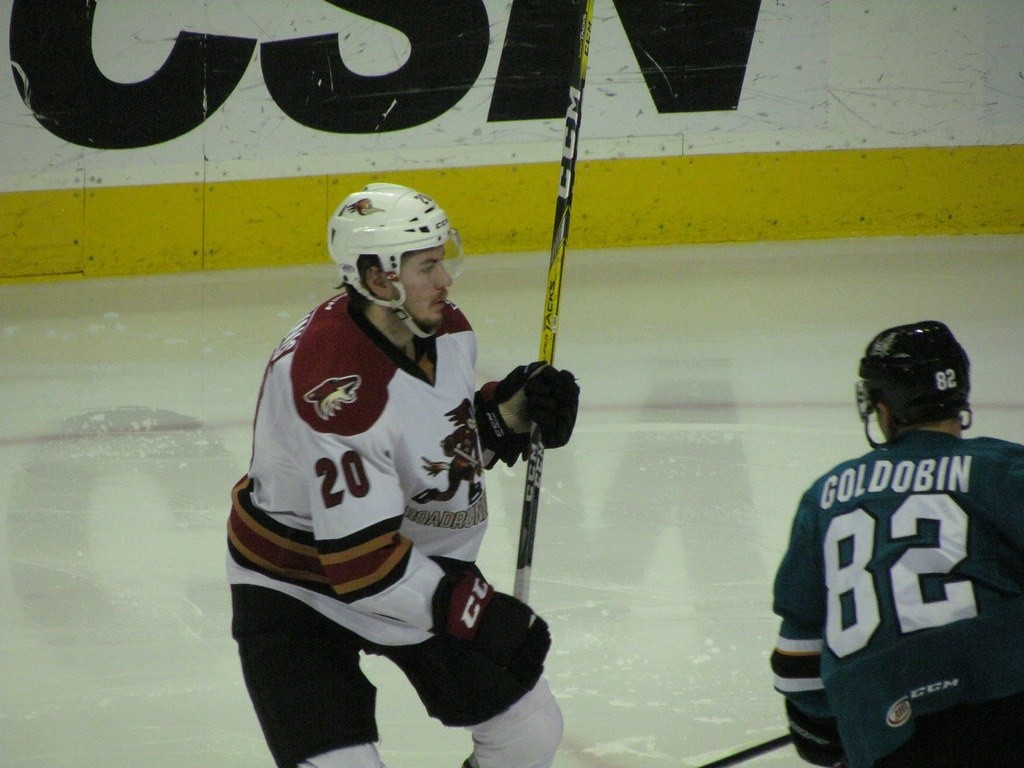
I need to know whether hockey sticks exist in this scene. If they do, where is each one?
[699,732,795,768]
[508,0,599,607]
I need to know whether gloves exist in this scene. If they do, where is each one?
[784,700,846,768]
[427,569,551,692]
[474,360,580,467]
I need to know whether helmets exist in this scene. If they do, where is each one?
[327,183,448,307]
[855,320,970,427]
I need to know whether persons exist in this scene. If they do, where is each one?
[770,320,1024,768]
[226,182,580,768]
